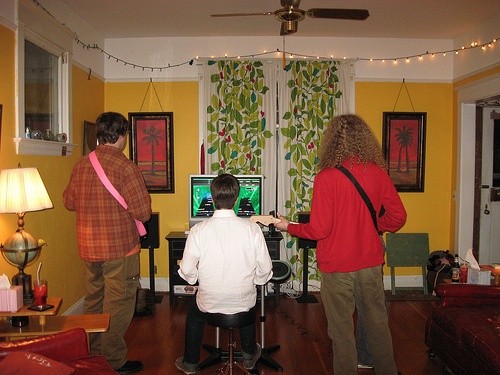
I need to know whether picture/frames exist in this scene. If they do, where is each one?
[383,111,425,192]
[128,111,174,193]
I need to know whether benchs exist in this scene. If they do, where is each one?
[424,284,500,375]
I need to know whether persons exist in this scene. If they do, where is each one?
[355,305,373,368]
[275,114,407,375]
[175,174,273,375]
[63,112,151,375]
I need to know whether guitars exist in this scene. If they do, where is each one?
[250,215,299,229]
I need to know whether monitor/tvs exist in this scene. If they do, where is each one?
[187,175,264,229]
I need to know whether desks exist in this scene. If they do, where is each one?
[165,231,282,304]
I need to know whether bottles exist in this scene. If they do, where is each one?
[452,254,460,284]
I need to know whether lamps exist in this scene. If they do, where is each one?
[0,163,53,229]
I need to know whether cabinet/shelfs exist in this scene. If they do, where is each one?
[0,298,110,354]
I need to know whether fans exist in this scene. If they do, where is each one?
[209,0,370,36]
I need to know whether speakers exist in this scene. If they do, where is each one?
[140,212,160,249]
[297,212,317,248]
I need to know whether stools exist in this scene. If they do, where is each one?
[206,309,256,375]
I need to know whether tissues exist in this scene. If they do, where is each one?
[464,248,491,285]
[0,274,24,314]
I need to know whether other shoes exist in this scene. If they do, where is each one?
[175,356,198,374]
[242,342,261,369]
[114,359,144,374]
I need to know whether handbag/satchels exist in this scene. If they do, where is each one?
[133,215,147,237]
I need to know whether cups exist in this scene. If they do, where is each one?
[460,263,468,284]
[33,280,48,307]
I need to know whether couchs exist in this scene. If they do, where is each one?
[0,328,120,375]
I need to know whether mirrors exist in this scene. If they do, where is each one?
[24,39,59,139]
